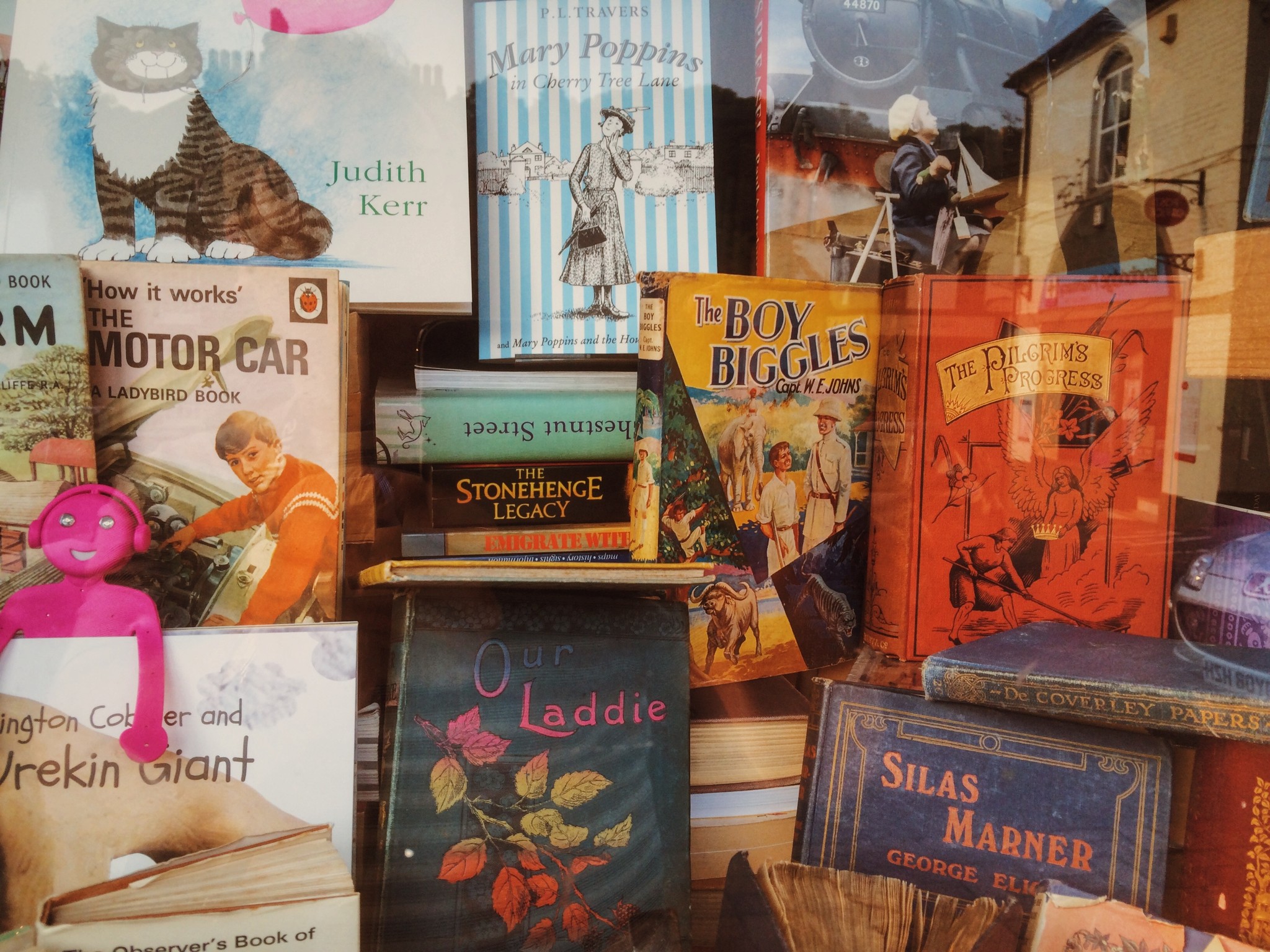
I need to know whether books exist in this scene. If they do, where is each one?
[1,0,1270,951]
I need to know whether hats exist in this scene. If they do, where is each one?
[888,94,920,140]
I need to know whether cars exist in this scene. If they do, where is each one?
[1172,527,1269,647]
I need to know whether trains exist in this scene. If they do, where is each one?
[763,0,1054,174]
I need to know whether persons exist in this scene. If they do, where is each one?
[888,93,994,276]
[160,412,337,627]
